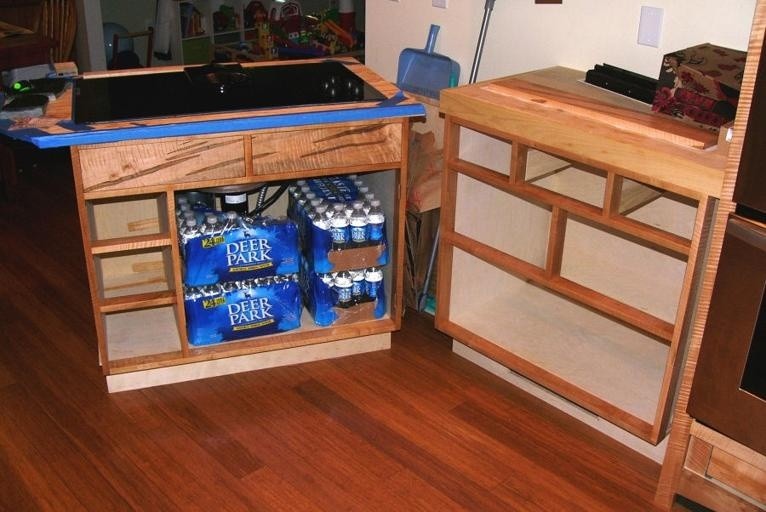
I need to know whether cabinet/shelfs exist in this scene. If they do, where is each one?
[171,0,272,64]
[0,51,425,397]
[433,66,728,450]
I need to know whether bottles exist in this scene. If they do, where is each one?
[174,174,389,347]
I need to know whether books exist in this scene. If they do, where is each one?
[181,4,205,36]
